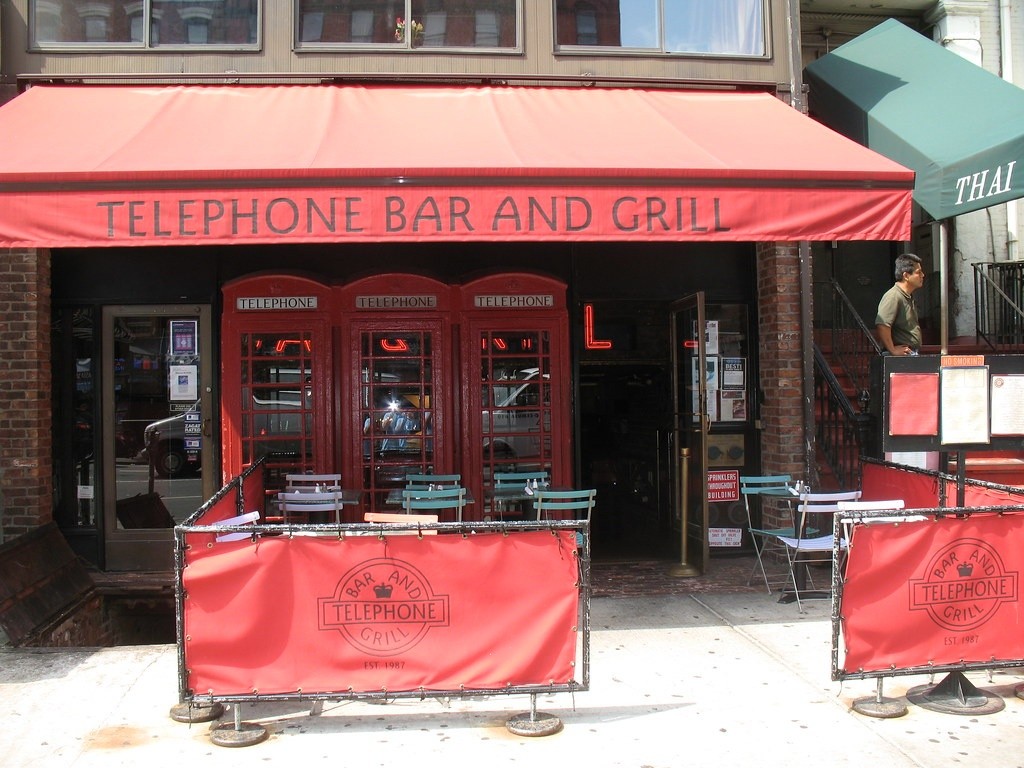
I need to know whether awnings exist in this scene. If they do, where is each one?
[0,84,915,249]
[803,17,1024,220]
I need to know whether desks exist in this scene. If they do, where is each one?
[270,487,360,524]
[758,486,851,605]
[384,487,476,537]
[482,484,575,532]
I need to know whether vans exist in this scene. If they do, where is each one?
[479,370,552,471]
[142,368,402,478]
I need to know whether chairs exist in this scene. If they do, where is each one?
[211,471,597,582]
[740,475,820,596]
[776,490,862,614]
[836,499,929,584]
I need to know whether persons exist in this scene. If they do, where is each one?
[874,254,925,356]
